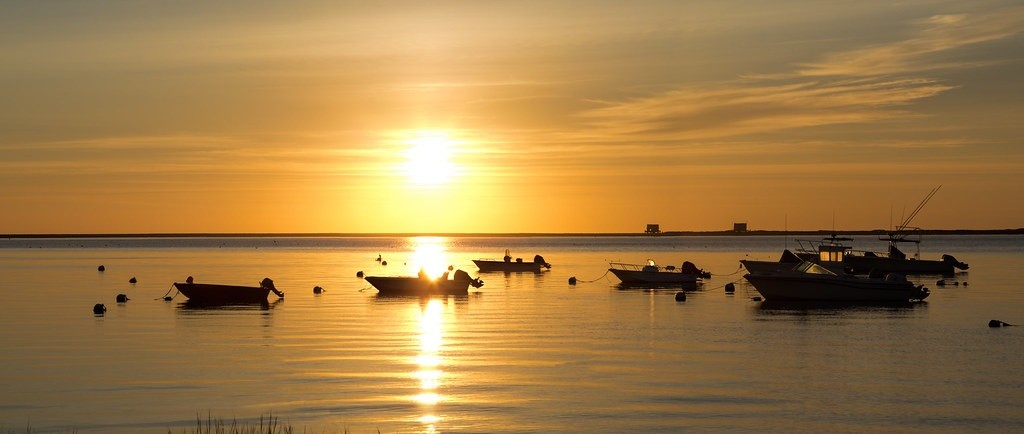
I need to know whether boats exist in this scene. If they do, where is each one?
[608,262,710,287]
[363,269,485,295]
[472,254,551,273]
[175,277,284,308]
[795,225,969,277]
[878,234,920,243]
[739,250,930,307]
